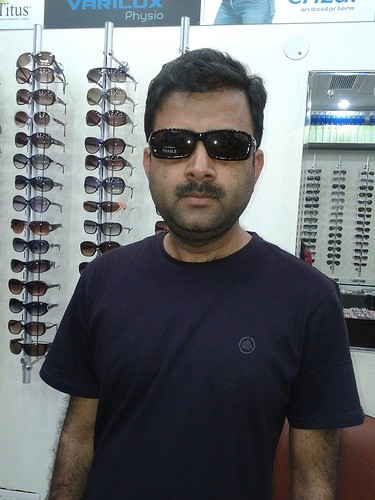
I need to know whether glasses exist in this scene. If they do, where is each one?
[148,128,257,160]
[8,52,137,358]
[300,168,374,283]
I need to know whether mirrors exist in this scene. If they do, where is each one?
[292,70,375,352]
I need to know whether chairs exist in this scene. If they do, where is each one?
[271,414,375,500]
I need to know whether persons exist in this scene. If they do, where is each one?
[213,0,275,25]
[38,49,364,500]
